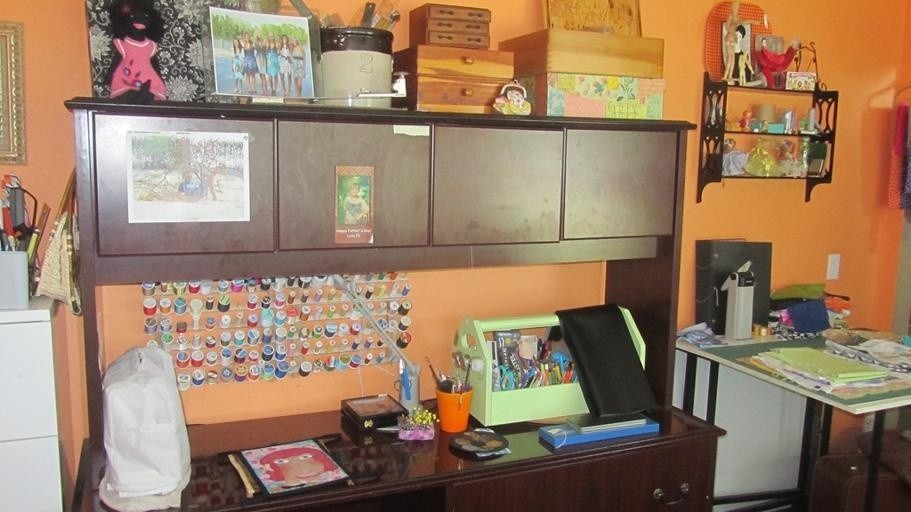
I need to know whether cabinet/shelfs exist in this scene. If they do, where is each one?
[431,115,681,247]
[62,96,435,257]
[1,20,28,166]
[697,71,840,202]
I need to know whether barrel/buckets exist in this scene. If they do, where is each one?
[320,26,394,110]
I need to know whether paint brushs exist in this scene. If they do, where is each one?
[465,358,472,389]
[425,356,441,387]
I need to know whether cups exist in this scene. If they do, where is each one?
[754,102,777,123]
[435,385,473,434]
[436,430,473,472]
[398,373,419,412]
[0,249,30,310]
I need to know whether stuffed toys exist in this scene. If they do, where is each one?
[104,0,169,101]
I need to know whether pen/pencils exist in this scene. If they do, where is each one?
[1,207,17,252]
[27,229,41,267]
[517,358,578,389]
[402,368,411,401]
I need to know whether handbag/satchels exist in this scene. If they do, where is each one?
[29,211,81,316]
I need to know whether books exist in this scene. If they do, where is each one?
[566,414,647,434]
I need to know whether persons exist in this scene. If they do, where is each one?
[177,171,202,196]
[723,110,806,178]
[259,447,338,486]
[343,183,369,224]
[231,30,306,97]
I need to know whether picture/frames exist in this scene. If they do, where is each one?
[197,1,327,108]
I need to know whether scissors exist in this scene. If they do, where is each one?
[446,375,458,393]
[394,379,411,391]
[498,365,514,391]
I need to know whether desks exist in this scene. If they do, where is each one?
[69,381,727,510]
[674,327,911,509]
